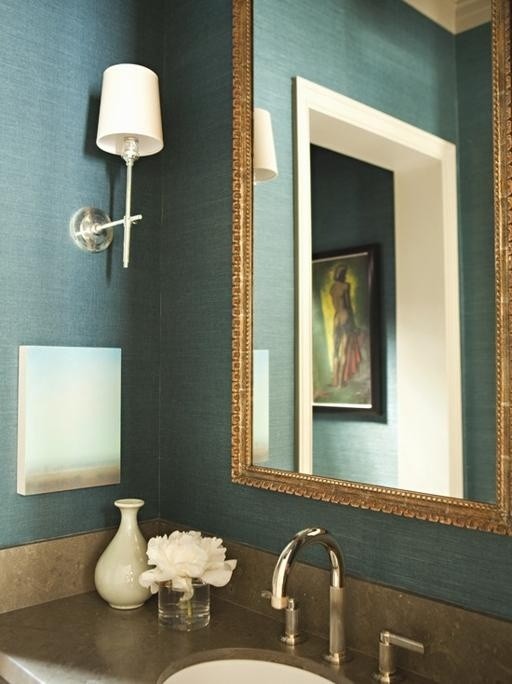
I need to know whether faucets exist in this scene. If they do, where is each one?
[270,527,356,665]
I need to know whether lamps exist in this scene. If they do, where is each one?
[253,106,280,182]
[68,63,166,268]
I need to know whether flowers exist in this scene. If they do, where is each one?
[140,530,238,616]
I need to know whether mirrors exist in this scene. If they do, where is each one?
[231,1,511,541]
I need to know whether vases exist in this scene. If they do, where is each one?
[157,583,211,632]
[93,498,151,611]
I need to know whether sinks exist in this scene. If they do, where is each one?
[157,647,353,684]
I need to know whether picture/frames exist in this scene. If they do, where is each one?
[312,244,386,419]
